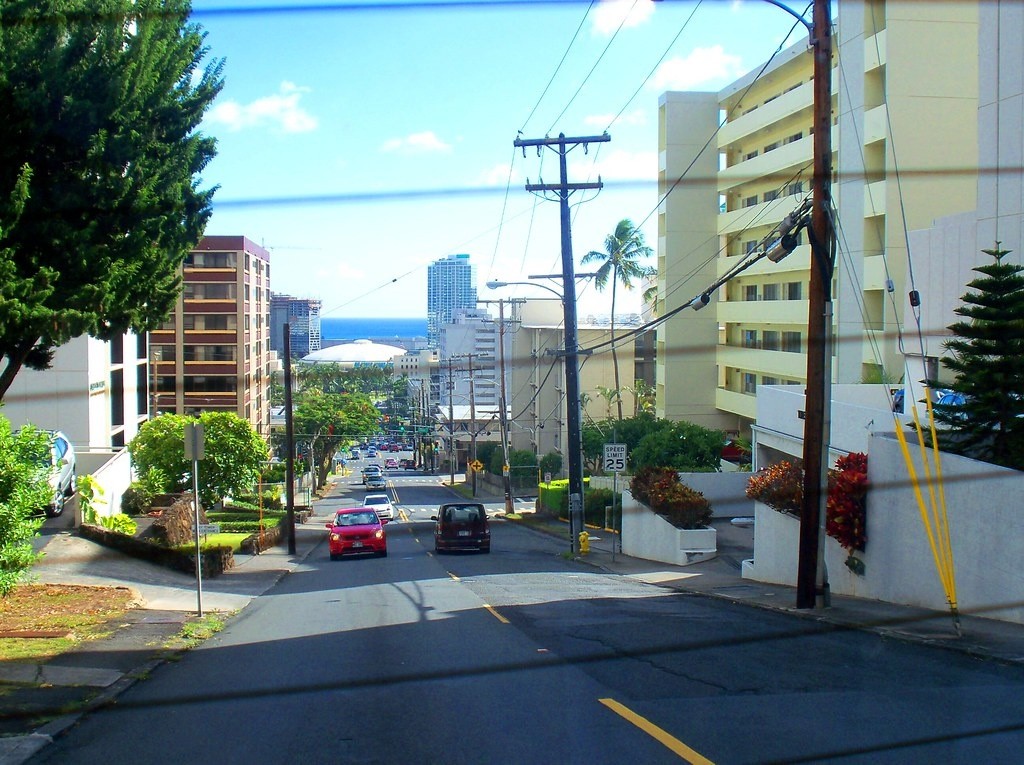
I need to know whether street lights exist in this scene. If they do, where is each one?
[442,393,479,497]
[461,378,516,517]
[486,279,585,553]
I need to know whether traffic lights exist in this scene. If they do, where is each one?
[435,442,439,452]
[400,426,405,436]
[296,441,303,464]
[341,445,345,453]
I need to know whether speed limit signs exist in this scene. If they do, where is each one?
[604,445,625,472]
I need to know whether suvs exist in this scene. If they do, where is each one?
[9,428,75,516]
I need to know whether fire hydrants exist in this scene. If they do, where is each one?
[577,531,591,555]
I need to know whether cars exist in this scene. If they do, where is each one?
[384,458,397,468]
[398,459,408,468]
[325,508,389,561]
[360,494,396,522]
[365,475,387,492]
[722,438,751,462]
[348,433,414,457]
[352,450,360,459]
[404,460,417,470]
[369,464,384,472]
[894,387,999,432]
[361,466,384,484]
[387,461,398,469]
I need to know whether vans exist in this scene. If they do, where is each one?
[430,503,492,554]
